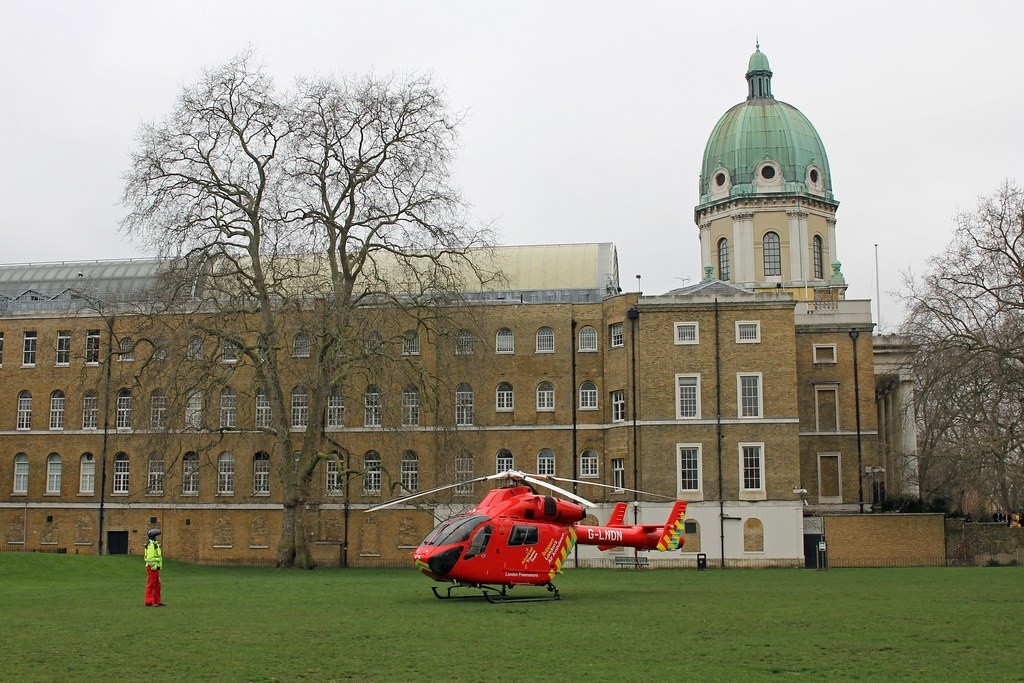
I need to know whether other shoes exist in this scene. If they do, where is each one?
[146,603,166,607]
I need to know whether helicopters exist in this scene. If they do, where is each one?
[365,467,691,606]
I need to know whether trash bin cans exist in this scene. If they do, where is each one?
[697,554,706,570]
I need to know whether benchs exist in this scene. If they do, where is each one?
[615,556,650,569]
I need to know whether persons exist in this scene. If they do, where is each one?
[993,508,1024,527]
[143,529,166,606]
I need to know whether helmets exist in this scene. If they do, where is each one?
[148,529,162,542]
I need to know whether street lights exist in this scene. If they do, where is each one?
[848,327,867,515]
[98,314,117,555]
[627,307,640,504]
